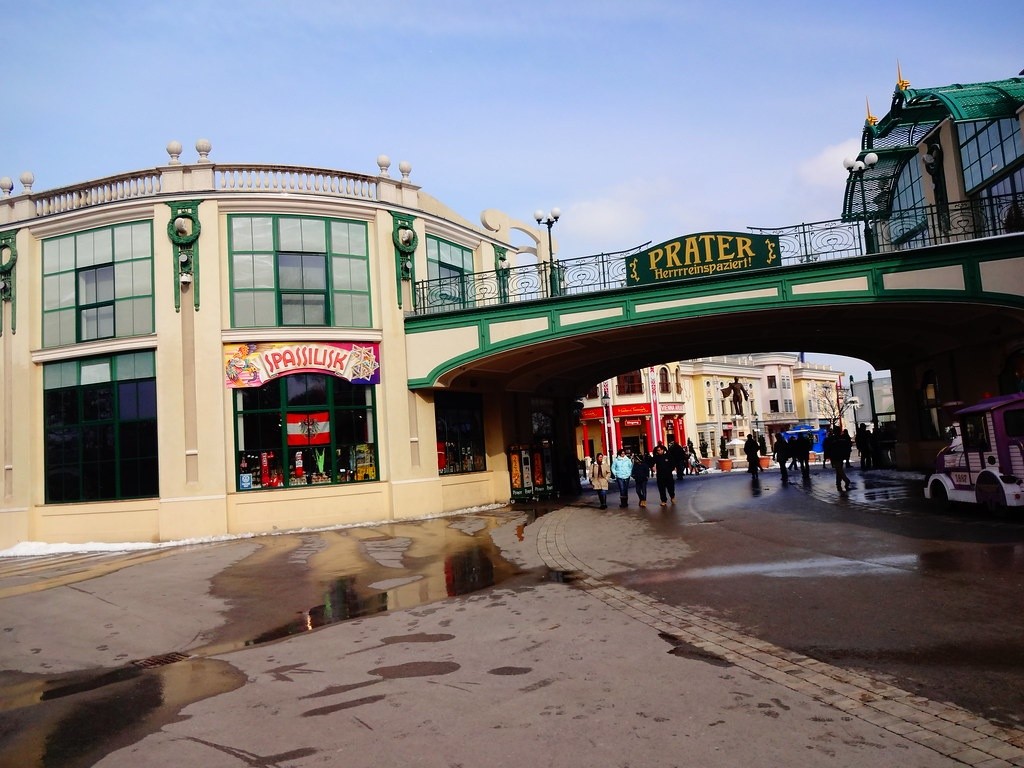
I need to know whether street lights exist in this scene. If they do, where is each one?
[601,391,616,478]
[842,152,877,254]
[533,207,561,297]
[754,413,760,445]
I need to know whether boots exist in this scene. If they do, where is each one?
[620,496,628,508]
[600,494,607,509]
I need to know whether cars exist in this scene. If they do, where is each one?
[922,394,1024,515]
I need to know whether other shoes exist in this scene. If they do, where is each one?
[640,500,646,507]
[660,501,666,506]
[836,483,841,486]
[671,498,675,505]
[844,481,850,488]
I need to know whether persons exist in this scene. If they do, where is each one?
[744,422,889,496]
[588,444,709,485]
[654,446,677,507]
[611,449,633,509]
[631,452,649,507]
[720,377,749,416]
[699,439,708,458]
[589,453,611,510]
